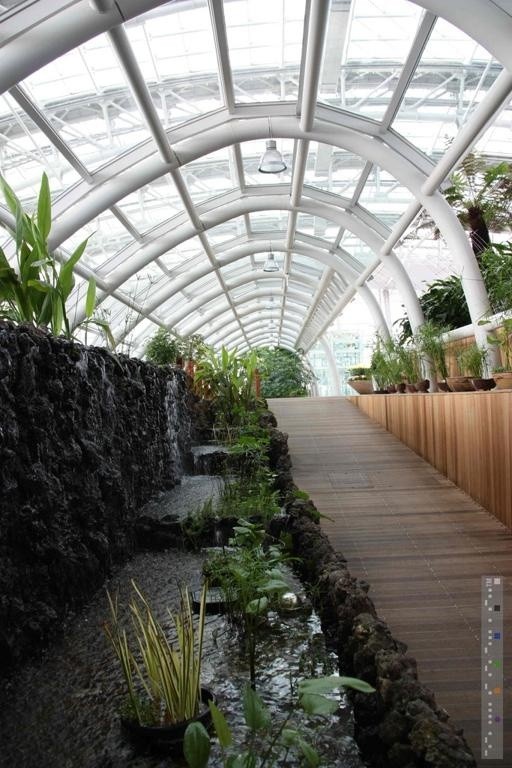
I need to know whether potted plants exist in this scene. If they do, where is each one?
[368,314,512,394]
[101,572,217,755]
[345,367,372,394]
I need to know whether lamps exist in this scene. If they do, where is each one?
[258,141,287,172]
[262,254,280,272]
